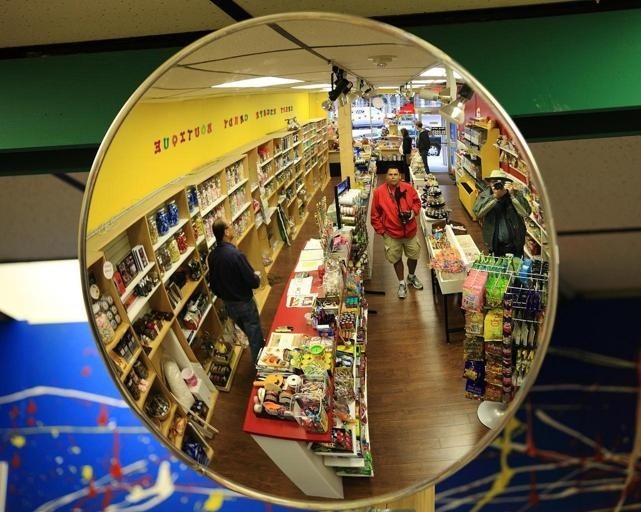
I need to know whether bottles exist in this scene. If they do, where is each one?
[142,159,250,277]
[93,305,117,345]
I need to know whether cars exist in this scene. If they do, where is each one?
[352,106,383,128]
[406,128,441,156]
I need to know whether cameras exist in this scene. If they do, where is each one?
[492,180,502,192]
[399,211,412,223]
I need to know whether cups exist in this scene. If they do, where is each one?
[181,368,197,385]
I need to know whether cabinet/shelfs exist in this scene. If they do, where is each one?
[86,106,548,502]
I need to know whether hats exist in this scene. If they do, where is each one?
[484,169,514,182]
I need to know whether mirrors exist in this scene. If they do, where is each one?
[77,12,559,502]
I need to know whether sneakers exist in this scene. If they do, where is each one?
[406,273,424,291]
[396,281,409,299]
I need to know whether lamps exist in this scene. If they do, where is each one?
[321,62,376,113]
[439,83,473,125]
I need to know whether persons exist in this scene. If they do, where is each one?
[472,169,533,256]
[400,128,413,183]
[416,120,432,175]
[371,164,425,299]
[207,217,266,365]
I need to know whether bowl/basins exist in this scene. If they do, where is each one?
[148,394,171,420]
